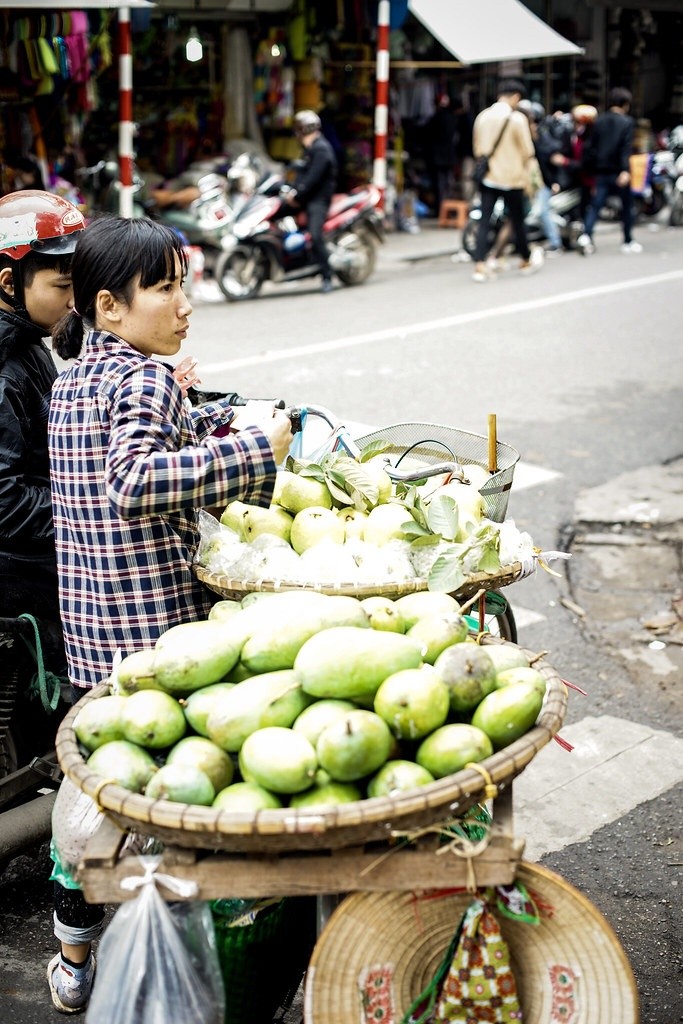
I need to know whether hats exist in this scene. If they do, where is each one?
[300,854,638,1024]
[497,78,528,95]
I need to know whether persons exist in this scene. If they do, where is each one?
[0,184,100,796]
[270,109,336,293]
[44,217,298,1021]
[471,77,648,284]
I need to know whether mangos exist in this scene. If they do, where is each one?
[70,590,547,813]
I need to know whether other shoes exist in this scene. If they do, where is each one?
[473,259,511,281]
[545,243,566,260]
[322,278,332,293]
[577,233,594,256]
[520,248,544,275]
[620,241,643,252]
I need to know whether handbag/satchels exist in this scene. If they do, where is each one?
[472,155,491,181]
[83,854,228,1024]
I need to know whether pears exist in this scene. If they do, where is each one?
[220,464,489,583]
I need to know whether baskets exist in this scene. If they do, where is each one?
[51,482,572,852]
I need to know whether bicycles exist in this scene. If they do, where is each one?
[209,389,515,946]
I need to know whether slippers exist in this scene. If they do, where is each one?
[46,947,96,1014]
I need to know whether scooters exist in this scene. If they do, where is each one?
[666,124,683,227]
[463,166,582,264]
[0,391,288,863]
[216,171,387,303]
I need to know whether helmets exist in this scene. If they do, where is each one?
[292,109,323,134]
[571,104,598,124]
[517,98,547,122]
[0,188,87,267]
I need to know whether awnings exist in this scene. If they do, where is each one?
[338,0,586,68]
